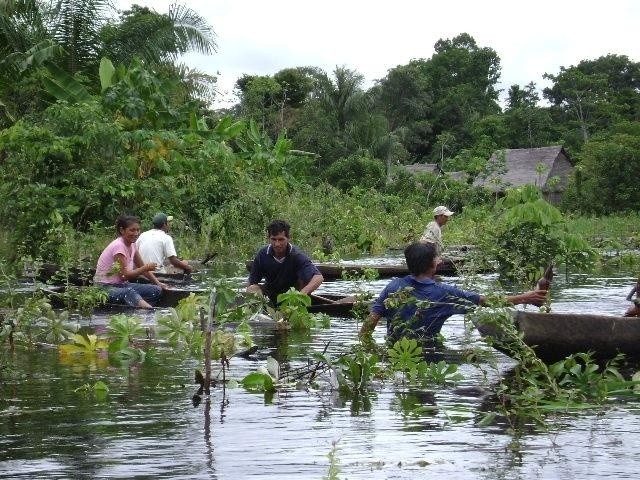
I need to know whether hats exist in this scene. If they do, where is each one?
[152,213,173,226]
[432,205,454,217]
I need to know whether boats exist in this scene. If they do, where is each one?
[464,309,640,367]
[312,256,498,281]
[38,284,375,317]
[31,262,196,289]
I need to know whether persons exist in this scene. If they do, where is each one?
[623,280,640,317]
[135,213,194,283]
[356,242,547,363]
[91,214,168,309]
[419,205,455,265]
[245,219,325,296]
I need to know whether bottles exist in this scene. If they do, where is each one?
[531,263,553,308]
